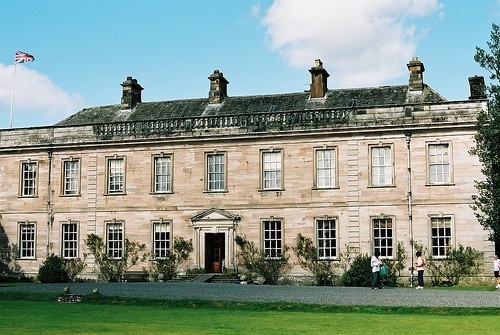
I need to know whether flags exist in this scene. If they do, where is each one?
[15,51,35,64]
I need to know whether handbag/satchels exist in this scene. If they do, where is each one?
[380,263,388,276]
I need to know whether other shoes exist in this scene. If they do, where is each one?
[416,286,424,289]
[496,283,500,288]
[372,286,385,290]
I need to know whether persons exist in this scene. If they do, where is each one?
[370,250,382,289]
[414,251,425,290]
[494,254,500,288]
